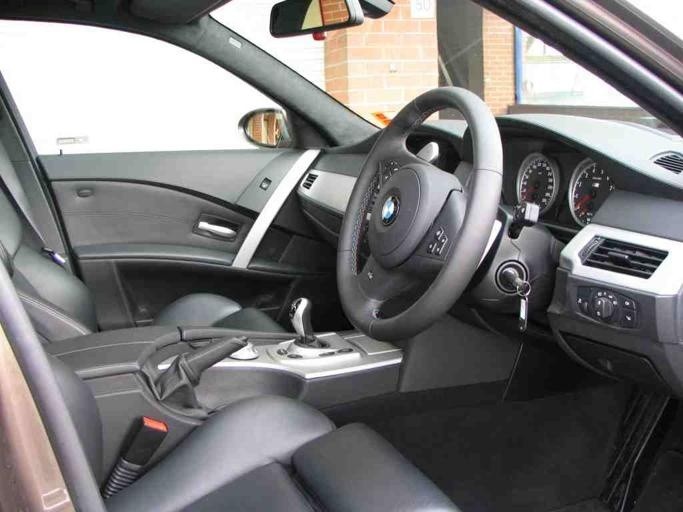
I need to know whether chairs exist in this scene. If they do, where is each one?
[0,325,463,512]
[0,201,289,348]
